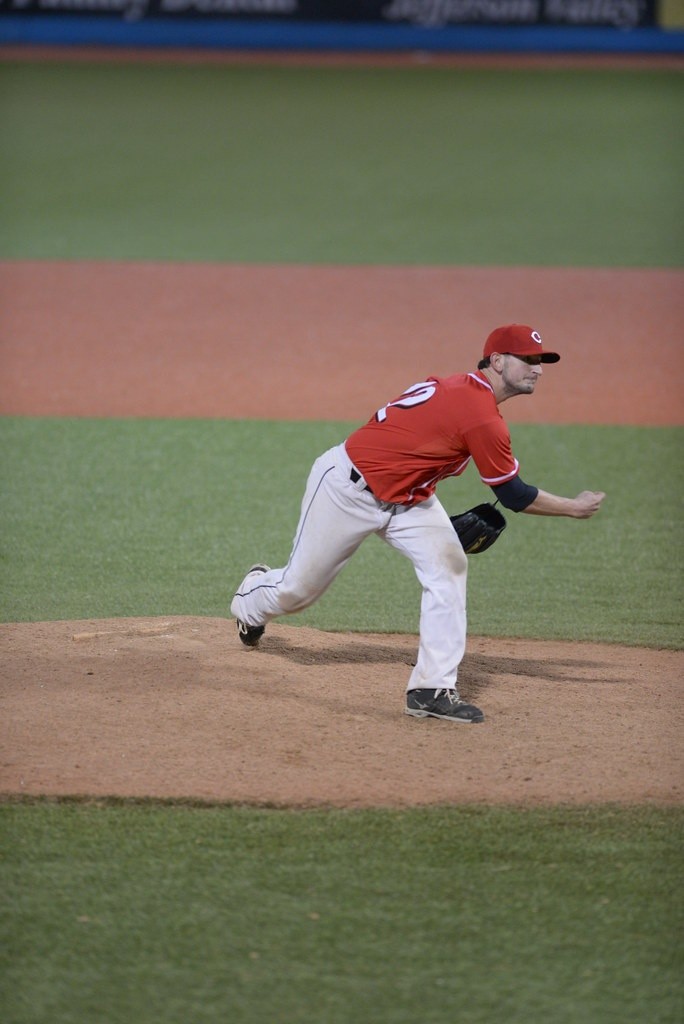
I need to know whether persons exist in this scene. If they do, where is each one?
[229,323,606,723]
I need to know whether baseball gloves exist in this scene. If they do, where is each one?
[449,502,507,555]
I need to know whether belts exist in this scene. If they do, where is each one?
[350,468,373,494]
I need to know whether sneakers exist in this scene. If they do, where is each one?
[237,564,270,646]
[405,688,484,722]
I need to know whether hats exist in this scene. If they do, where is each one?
[483,323,560,363]
